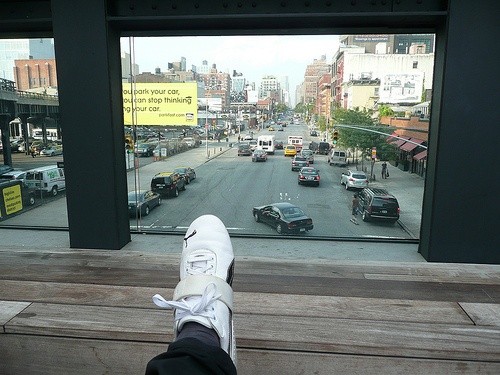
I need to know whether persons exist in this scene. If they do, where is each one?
[145,214,237,375]
[352,193,358,221]
[381,162,387,179]
[30,147,36,158]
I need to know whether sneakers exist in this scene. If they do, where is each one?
[153,214,238,368]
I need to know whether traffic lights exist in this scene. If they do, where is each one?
[332,130,339,145]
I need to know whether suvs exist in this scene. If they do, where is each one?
[150,170,187,198]
[355,186,400,224]
[291,153,311,171]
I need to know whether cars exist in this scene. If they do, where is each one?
[288,136,331,164]
[252,202,314,234]
[252,149,268,162]
[0,135,65,217]
[284,145,297,157]
[124,112,225,156]
[275,140,284,149]
[128,189,161,217]
[172,166,196,184]
[298,166,321,186]
[237,130,257,156]
[310,131,317,136]
[264,111,299,130]
[340,169,369,190]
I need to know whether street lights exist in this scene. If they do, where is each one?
[206,84,225,159]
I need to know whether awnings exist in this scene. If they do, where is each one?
[386,134,427,161]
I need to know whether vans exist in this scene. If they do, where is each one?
[257,134,276,155]
[328,147,348,167]
